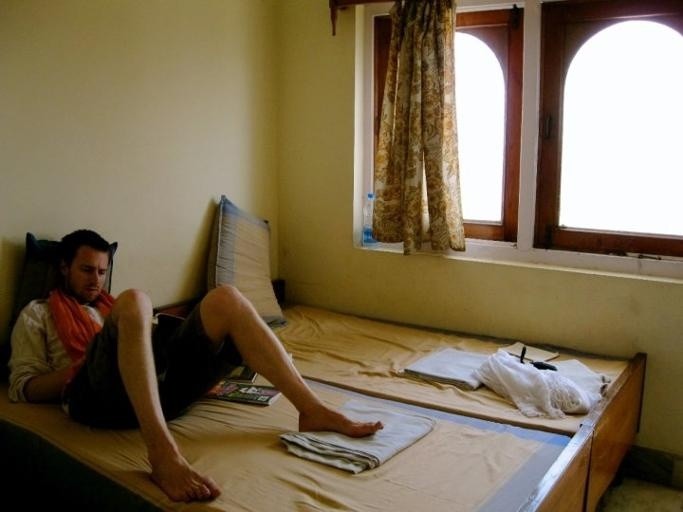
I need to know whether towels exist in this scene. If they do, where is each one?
[279,396,436,474]
[404,346,493,392]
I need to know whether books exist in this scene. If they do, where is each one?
[207,378,281,406]
[152,314,184,343]
[504,342,559,362]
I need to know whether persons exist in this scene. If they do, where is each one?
[7,228,383,501]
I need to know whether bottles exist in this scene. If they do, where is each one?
[363,193,377,246]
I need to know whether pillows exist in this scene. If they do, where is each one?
[210,192,292,327]
[20,229,121,308]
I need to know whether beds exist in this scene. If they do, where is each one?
[0,299,648,512]
